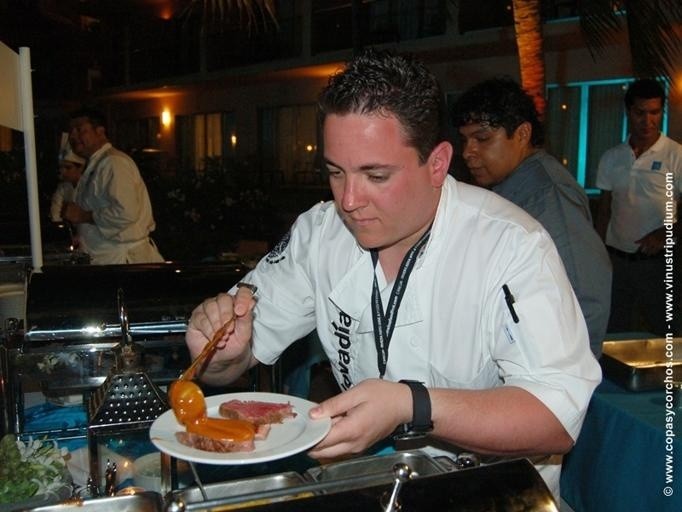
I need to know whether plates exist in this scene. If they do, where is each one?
[148,388,335,468]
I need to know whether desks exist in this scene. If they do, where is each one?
[561,329,680,511]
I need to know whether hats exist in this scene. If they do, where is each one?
[60,132,87,165]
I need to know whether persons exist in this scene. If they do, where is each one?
[50,160,87,244]
[185,49,603,511]
[447,74,613,365]
[594,78,682,337]
[61,110,166,263]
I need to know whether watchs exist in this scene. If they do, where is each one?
[394,378,434,442]
